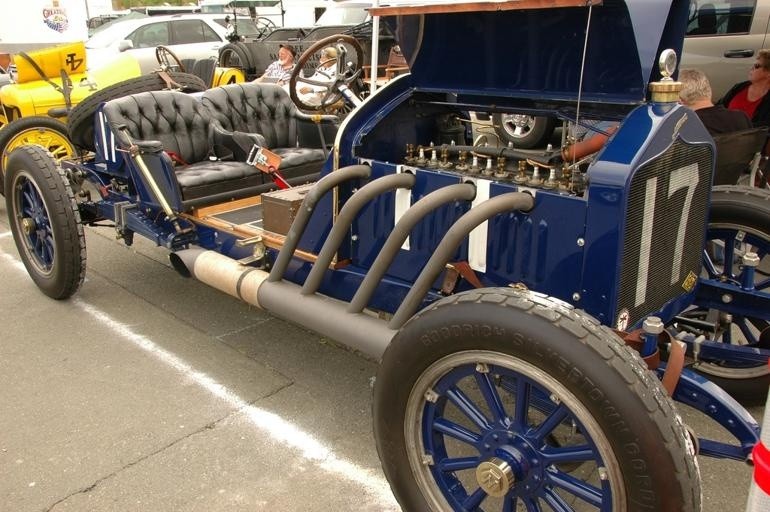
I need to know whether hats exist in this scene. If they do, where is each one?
[278,43,297,57]
[320,46,338,60]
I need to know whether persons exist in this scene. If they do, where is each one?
[280,45,339,106]
[715,47,769,170]
[674,67,755,187]
[251,42,304,88]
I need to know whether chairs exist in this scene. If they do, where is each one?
[102,82,340,202]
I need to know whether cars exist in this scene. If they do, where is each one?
[0,0,768,509]
[7,9,268,89]
[1,47,259,196]
